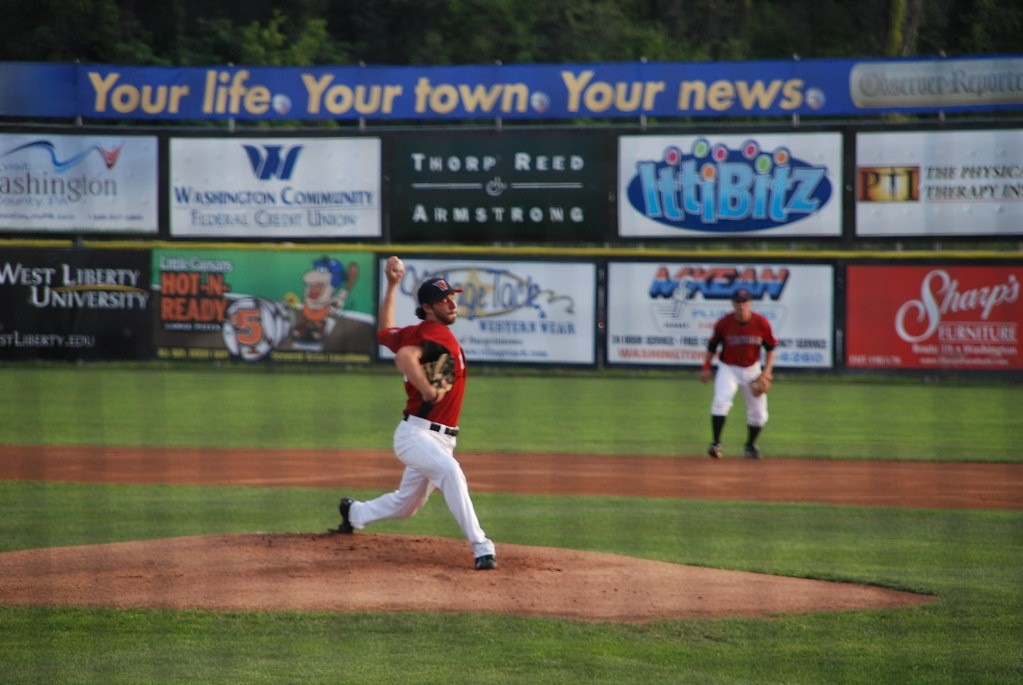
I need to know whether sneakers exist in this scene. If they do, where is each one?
[475,555,496,569]
[337,497,354,535]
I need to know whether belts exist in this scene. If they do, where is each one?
[403,415,459,437]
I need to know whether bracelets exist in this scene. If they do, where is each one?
[430,387,439,404]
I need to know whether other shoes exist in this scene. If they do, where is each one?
[707,443,722,458]
[745,444,762,458]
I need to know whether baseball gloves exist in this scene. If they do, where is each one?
[422,354,455,402]
[749,373,772,394]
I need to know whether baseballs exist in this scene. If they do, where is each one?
[394,260,404,272]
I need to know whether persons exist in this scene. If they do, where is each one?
[700,289,777,458]
[338,256,500,573]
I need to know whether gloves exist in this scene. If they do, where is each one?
[699,363,712,383]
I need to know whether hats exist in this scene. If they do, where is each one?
[732,289,750,303]
[417,278,464,306]
